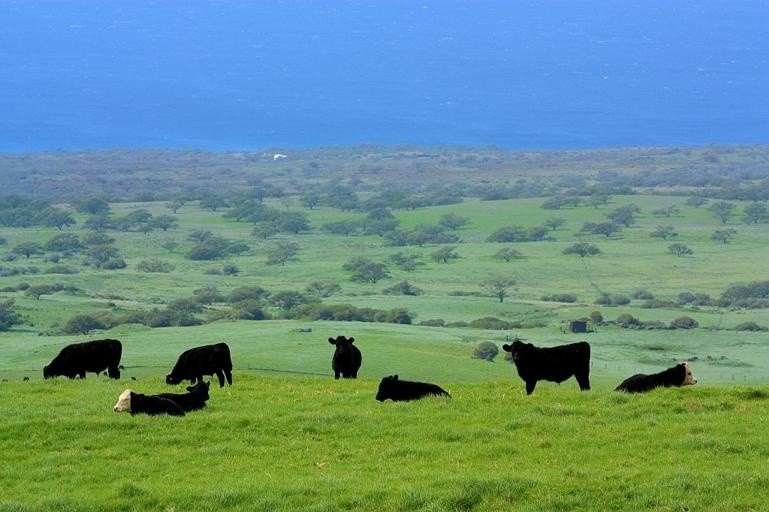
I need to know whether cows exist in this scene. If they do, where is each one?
[614,361,697,395]
[43,339,122,380]
[166,343,232,388]
[113,388,186,417]
[503,341,590,395]
[376,375,453,402]
[157,381,210,413]
[328,336,362,379]
